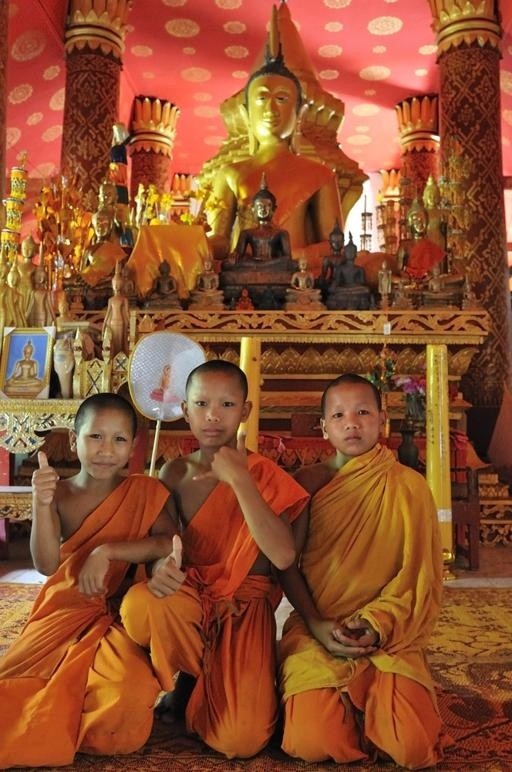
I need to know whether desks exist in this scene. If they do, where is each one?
[0,400,82,523]
[130,309,492,580]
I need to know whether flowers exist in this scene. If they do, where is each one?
[364,353,426,429]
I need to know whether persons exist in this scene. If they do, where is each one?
[102,277,130,352]
[194,257,220,292]
[119,360,311,760]
[7,344,42,387]
[395,181,466,290]
[144,261,180,300]
[377,261,392,296]
[0,393,181,769]
[287,256,322,294]
[205,62,397,286]
[317,227,379,311]
[221,188,300,282]
[271,374,456,770]
[0,183,131,326]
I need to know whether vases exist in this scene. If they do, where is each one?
[397,429,419,469]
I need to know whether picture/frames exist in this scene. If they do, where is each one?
[1,325,56,400]
